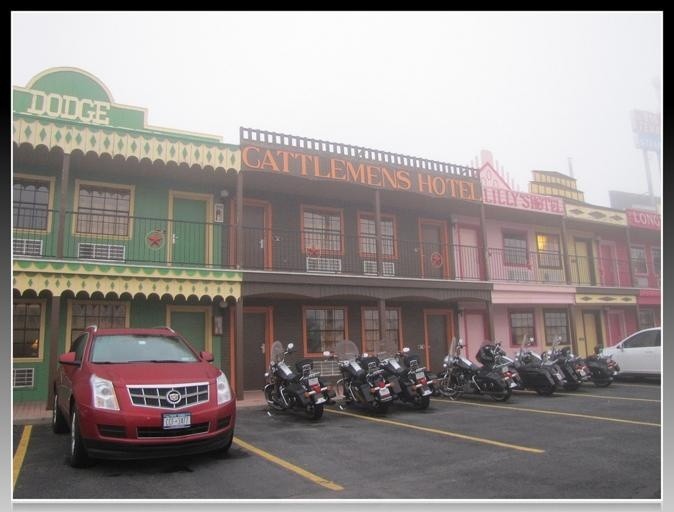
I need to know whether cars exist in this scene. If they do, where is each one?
[597,327,660,376]
[51,325,236,468]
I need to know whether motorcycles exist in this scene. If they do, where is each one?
[322,340,398,414]
[263,340,336,420]
[373,347,441,409]
[437,335,621,403]
